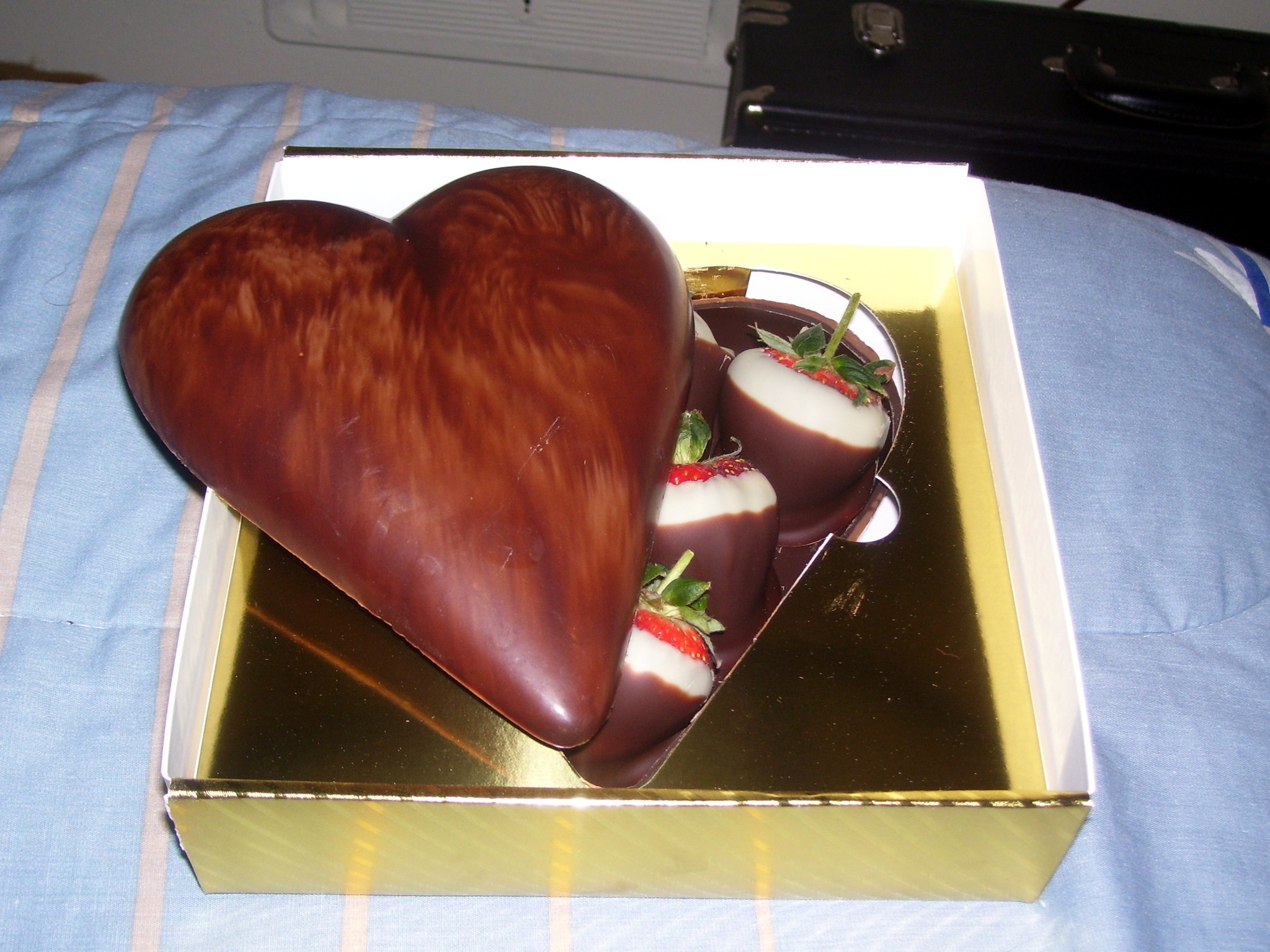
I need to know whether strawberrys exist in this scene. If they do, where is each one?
[583,291,889,769]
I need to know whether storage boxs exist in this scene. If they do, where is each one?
[160,145,1098,903]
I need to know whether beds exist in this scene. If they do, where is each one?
[0,74,1270,952]
